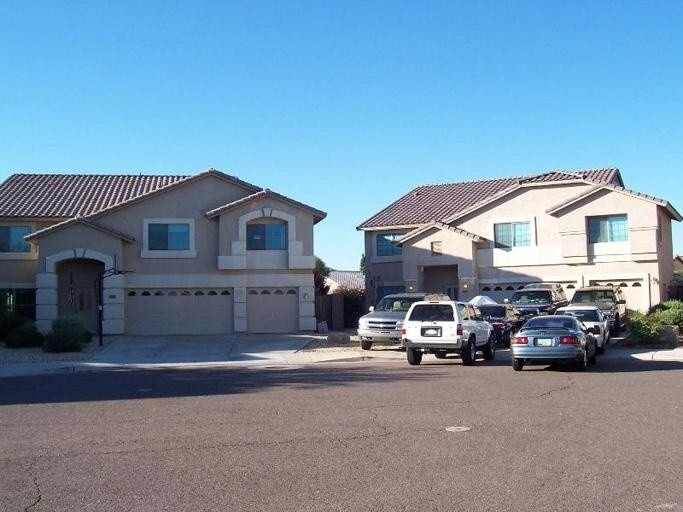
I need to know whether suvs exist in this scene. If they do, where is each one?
[356,292,451,350]
[566,284,629,336]
[503,282,569,318]
[399,299,497,367]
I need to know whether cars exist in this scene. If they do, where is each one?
[552,303,610,354]
[505,313,600,373]
[477,301,530,349]
